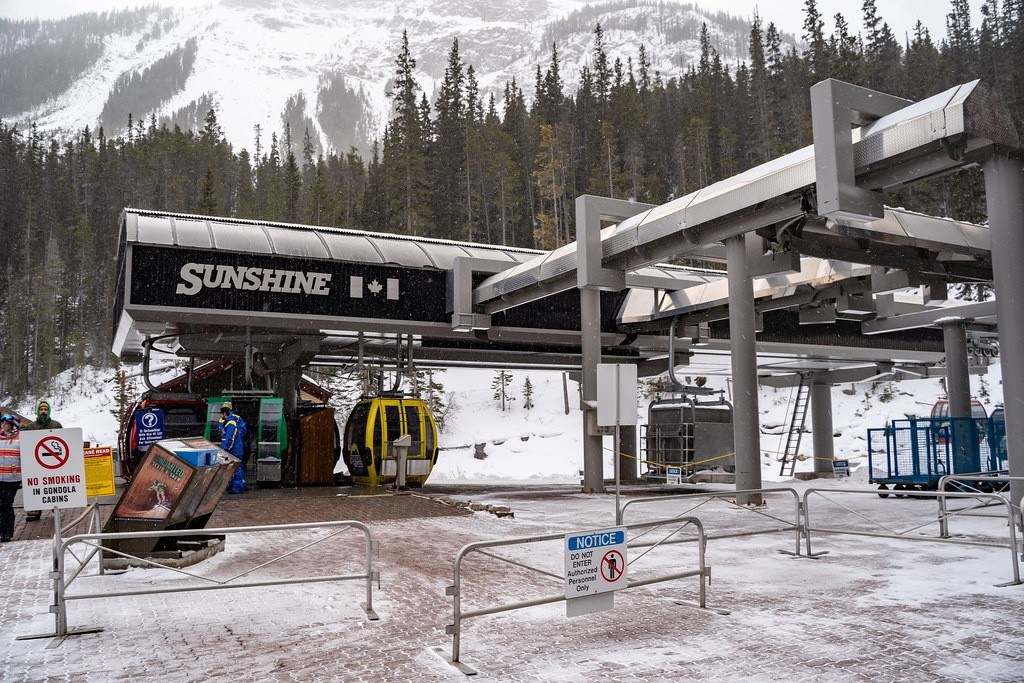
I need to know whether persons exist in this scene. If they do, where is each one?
[0,414,24,542]
[26,401,63,521]
[215,402,249,492]
[219,407,246,494]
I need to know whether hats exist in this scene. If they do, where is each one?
[40,402,48,408]
[223,401,232,411]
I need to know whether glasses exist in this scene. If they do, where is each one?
[0,413,13,421]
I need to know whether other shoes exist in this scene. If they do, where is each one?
[0,535,11,541]
[26,516,40,522]
[229,490,237,494]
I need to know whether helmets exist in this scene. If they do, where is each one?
[9,415,20,429]
